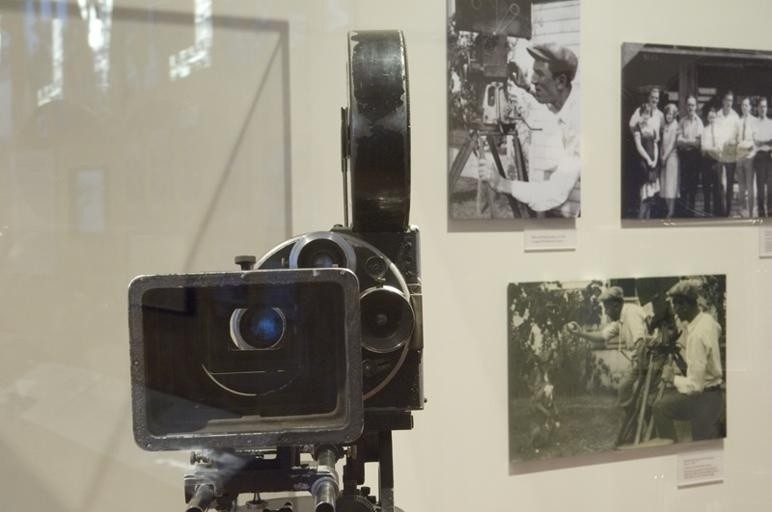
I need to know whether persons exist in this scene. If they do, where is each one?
[507,279,726,454]
[627,85,771,220]
[477,43,581,219]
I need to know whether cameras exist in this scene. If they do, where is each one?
[124,30,427,511]
[633,277,684,344]
[463,35,509,83]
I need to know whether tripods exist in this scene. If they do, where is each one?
[449,120,536,220]
[615,346,687,451]
